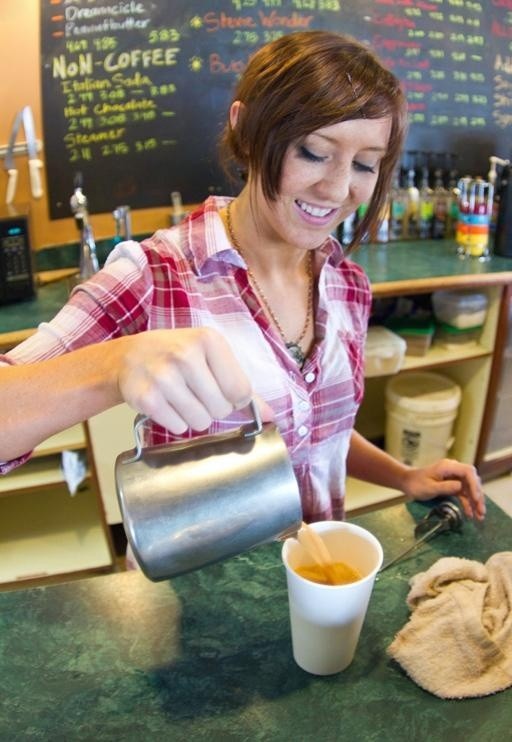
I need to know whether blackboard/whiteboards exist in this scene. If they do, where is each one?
[39,0,512,221]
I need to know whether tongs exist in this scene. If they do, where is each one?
[3,108,45,205]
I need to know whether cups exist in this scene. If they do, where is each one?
[281,520,384,676]
[452,212,490,259]
[114,396,302,582]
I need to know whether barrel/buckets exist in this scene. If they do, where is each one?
[384,371,462,468]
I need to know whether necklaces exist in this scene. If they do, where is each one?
[226,198,311,371]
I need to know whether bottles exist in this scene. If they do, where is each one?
[340,149,459,244]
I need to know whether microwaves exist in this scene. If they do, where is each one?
[0,202,39,306]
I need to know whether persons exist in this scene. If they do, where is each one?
[1,31,486,572]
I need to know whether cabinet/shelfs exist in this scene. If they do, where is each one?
[0,241,512,585]
